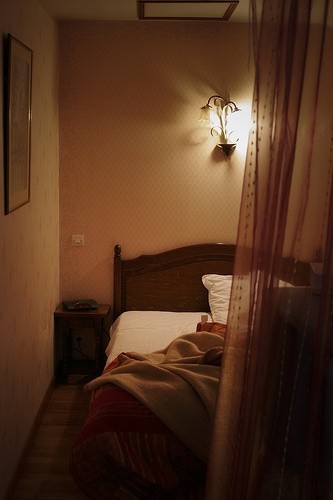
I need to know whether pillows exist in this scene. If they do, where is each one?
[202,274,295,325]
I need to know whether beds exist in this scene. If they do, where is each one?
[68,242,311,500]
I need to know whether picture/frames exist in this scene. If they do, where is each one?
[5,34,33,215]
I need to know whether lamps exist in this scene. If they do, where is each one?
[199,95,242,161]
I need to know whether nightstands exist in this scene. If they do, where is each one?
[54,302,111,376]
